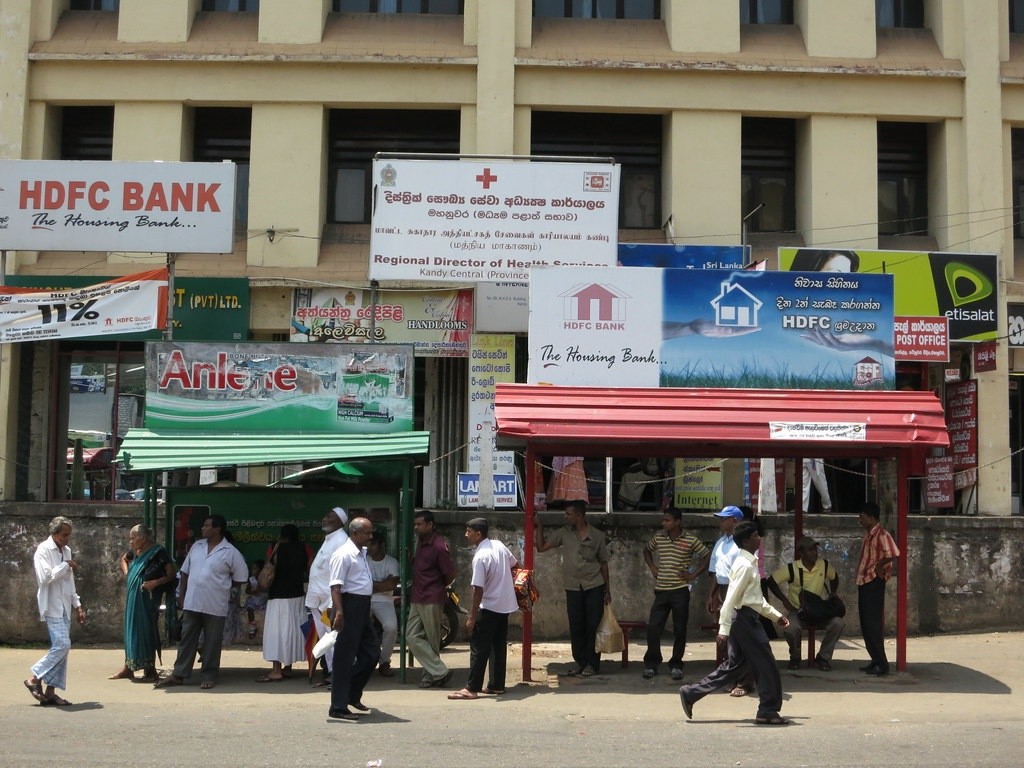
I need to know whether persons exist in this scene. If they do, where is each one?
[304,506,400,720]
[643,507,712,680]
[790,458,831,514]
[155,514,249,689]
[617,458,663,510]
[23,516,84,706]
[706,505,775,697]
[855,504,900,675]
[546,457,590,506]
[449,518,519,700]
[247,523,315,682]
[108,524,175,684]
[533,500,612,676]
[405,510,455,688]
[766,536,844,672]
[679,520,790,725]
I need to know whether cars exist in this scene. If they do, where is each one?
[66,447,162,502]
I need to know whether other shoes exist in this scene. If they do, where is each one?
[817,653,832,671]
[860,664,889,674]
[643,666,658,678]
[153,675,183,686]
[789,658,799,669]
[418,670,453,688]
[380,663,393,675]
[201,680,215,689]
[671,668,683,679]
[329,703,369,720]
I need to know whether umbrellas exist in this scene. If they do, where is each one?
[301,614,321,685]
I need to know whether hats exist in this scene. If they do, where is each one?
[331,507,348,526]
[799,536,820,549]
[713,505,744,520]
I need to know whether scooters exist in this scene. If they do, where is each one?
[375,578,470,648]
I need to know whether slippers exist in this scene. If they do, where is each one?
[256,675,283,682]
[24,680,72,706]
[449,689,506,699]
[823,507,832,514]
[731,688,748,697]
[568,666,595,676]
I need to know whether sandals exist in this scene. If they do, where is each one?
[756,714,789,724]
[680,689,692,719]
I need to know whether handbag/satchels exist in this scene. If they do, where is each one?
[798,590,845,625]
[595,602,625,654]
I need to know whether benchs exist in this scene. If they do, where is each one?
[702,624,826,667]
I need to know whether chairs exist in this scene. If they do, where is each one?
[618,621,646,667]
